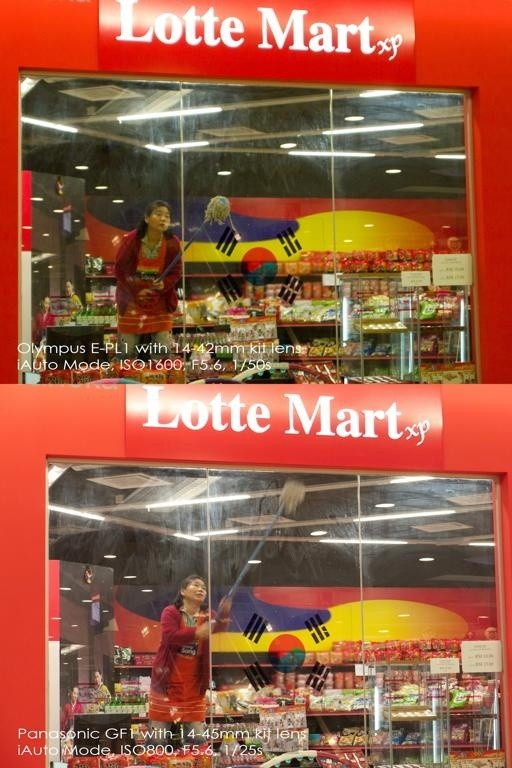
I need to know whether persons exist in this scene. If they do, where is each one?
[114,199,183,360]
[60,668,111,756]
[146,574,233,751]
[32,280,83,359]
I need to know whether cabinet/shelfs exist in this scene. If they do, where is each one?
[41,271,475,386]
[64,661,505,768]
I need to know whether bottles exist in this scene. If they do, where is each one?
[77,303,117,325]
[105,694,149,717]
